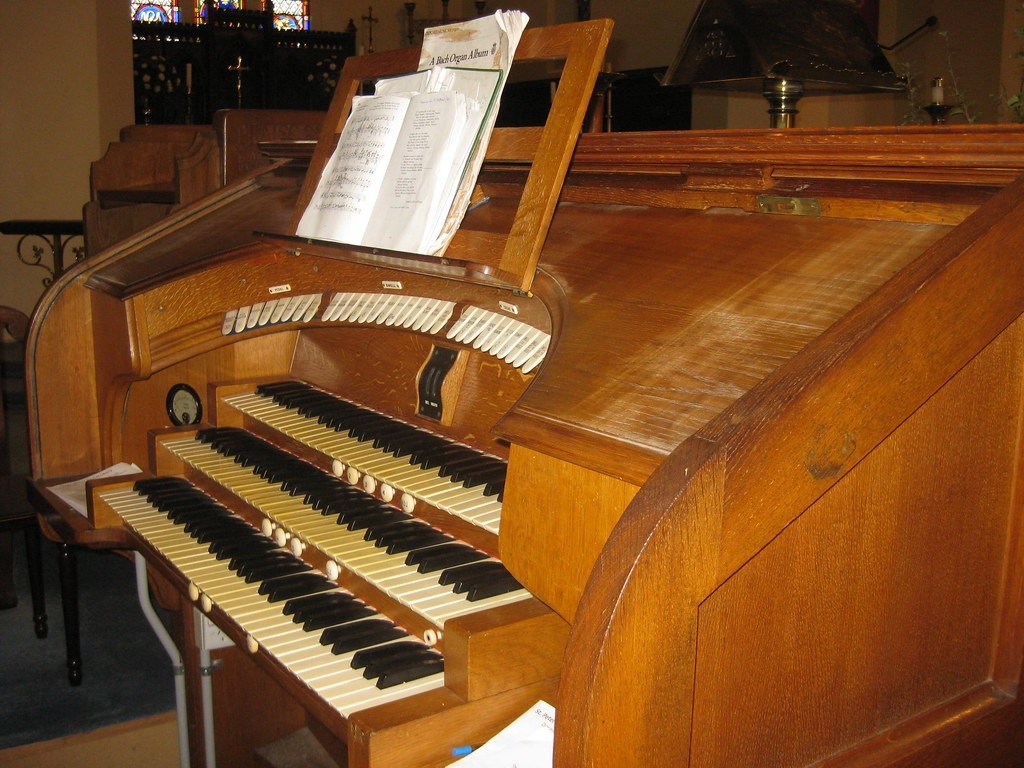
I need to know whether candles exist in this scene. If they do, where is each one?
[186,63,192,93]
[931,78,945,105]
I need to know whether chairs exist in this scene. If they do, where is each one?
[0,304,86,684]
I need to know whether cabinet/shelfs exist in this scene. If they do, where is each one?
[84,125,222,258]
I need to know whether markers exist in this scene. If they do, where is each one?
[452,743,481,756]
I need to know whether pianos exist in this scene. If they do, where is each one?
[21,120,1024,768]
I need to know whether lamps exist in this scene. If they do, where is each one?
[658,0,904,128]
